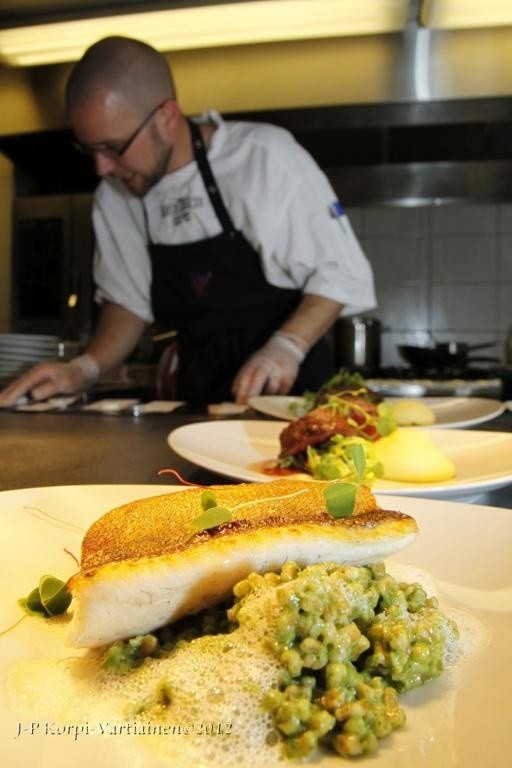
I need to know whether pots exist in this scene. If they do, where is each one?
[397,339,501,376]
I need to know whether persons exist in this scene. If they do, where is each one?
[0,35,379,409]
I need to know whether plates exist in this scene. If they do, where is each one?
[0,479,508,768]
[165,415,510,499]
[246,390,506,434]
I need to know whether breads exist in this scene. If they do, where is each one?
[64,480,420,651]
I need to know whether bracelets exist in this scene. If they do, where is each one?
[270,335,305,363]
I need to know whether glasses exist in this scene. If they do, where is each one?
[66,93,181,162]
